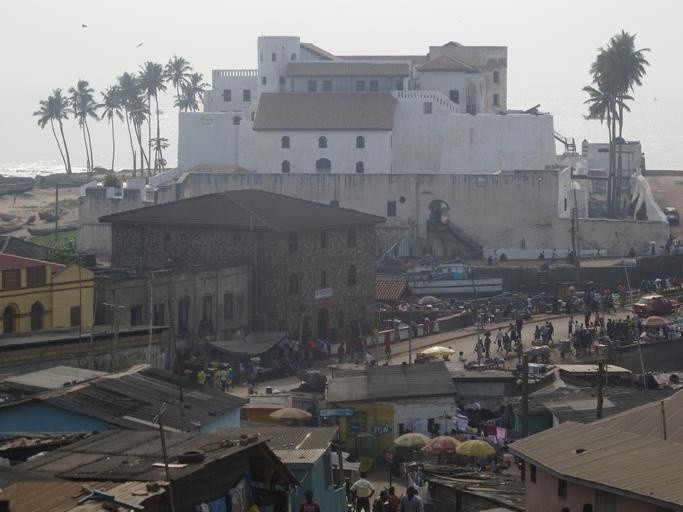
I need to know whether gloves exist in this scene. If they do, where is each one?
[0,173,37,200]
[401,264,503,298]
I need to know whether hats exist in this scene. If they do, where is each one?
[633,294,678,316]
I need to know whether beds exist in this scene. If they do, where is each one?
[392,432,430,448]
[455,439,497,471]
[421,435,461,464]
[269,406,311,421]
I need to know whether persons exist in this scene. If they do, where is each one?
[458,351,466,367]
[583,503,592,512]
[560,507,569,512]
[429,234,682,264]
[393,317,440,342]
[349,472,375,512]
[371,482,423,511]
[474,275,683,363]
[195,333,390,390]
[299,490,320,511]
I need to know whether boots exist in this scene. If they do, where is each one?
[662,207,679,225]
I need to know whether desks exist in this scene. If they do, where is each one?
[405,294,433,369]
[595,343,608,420]
